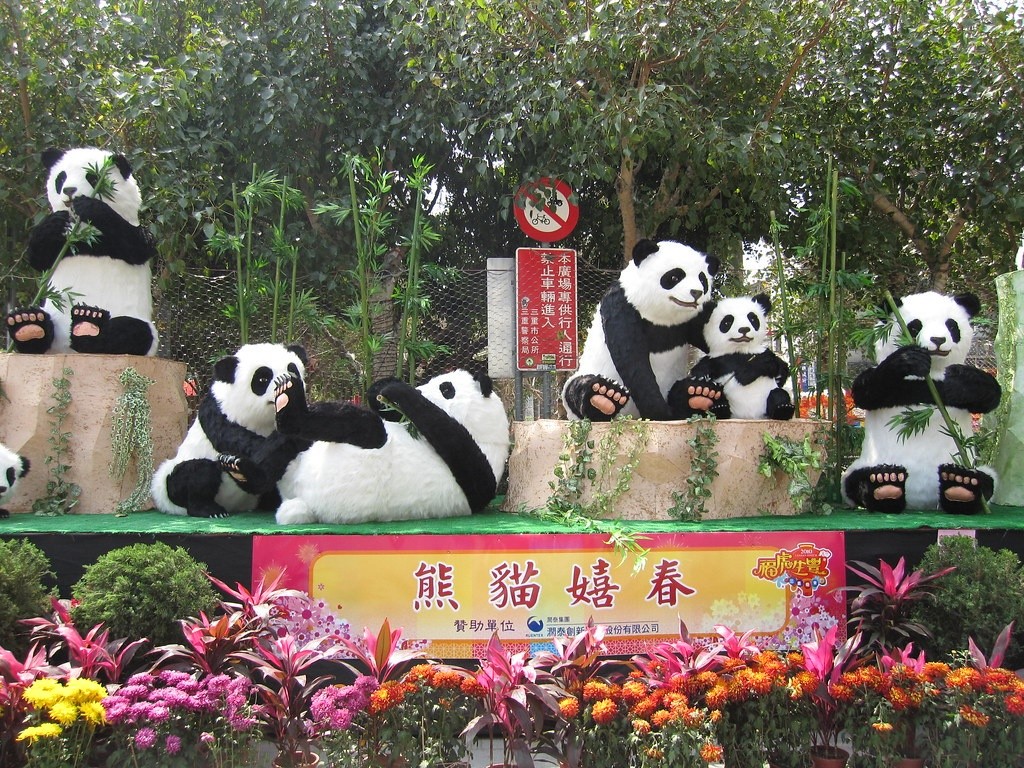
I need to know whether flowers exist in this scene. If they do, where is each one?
[1,556,1024,764]
